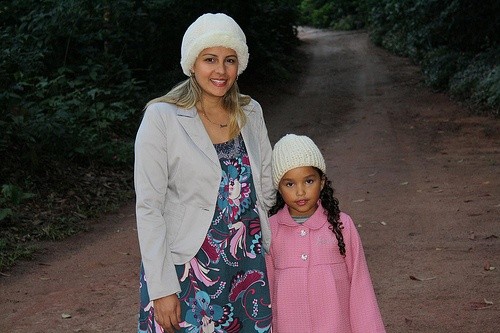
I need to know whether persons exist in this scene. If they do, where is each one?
[264,134,387,333]
[134,13,278,333]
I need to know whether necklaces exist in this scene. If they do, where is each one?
[203,114,232,128]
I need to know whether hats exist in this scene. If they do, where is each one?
[180,13,249,77]
[271,134,327,191]
